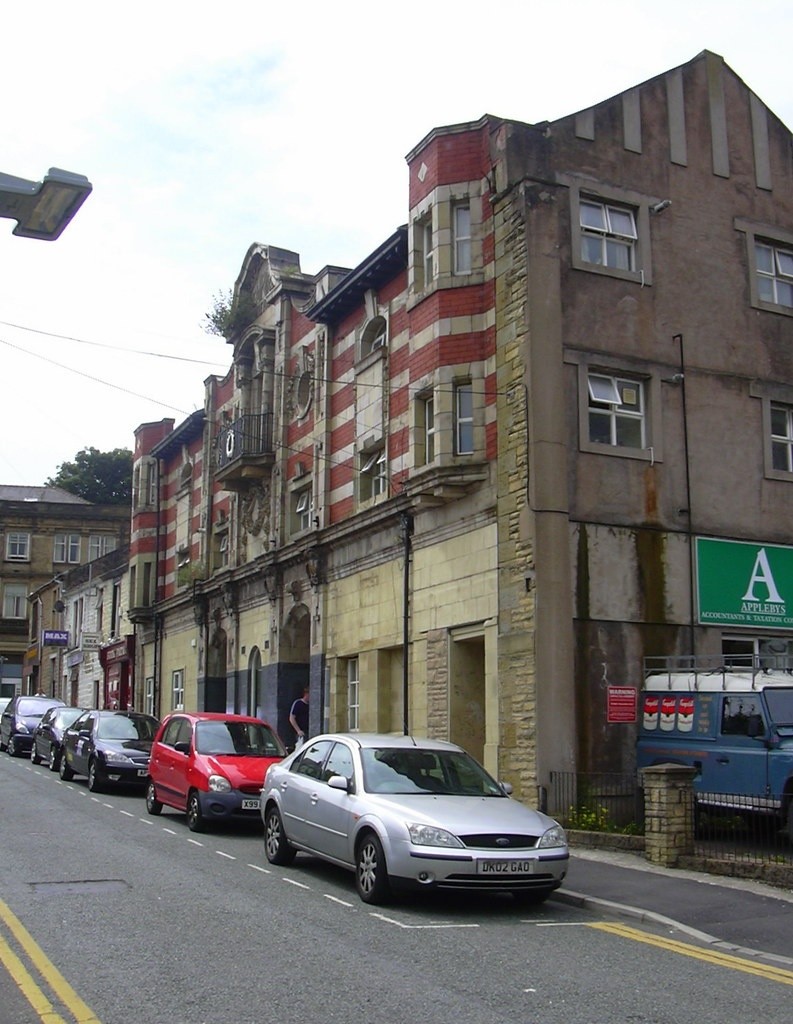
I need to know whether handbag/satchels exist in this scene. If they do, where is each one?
[295,736,304,751]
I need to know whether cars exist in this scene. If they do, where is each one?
[260,732,571,908]
[0,696,69,756]
[146,712,291,837]
[30,707,101,771]
[61,709,168,793]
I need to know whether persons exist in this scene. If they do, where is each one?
[34,688,47,697]
[289,687,309,752]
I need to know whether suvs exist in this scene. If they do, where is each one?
[636,669,793,843]
